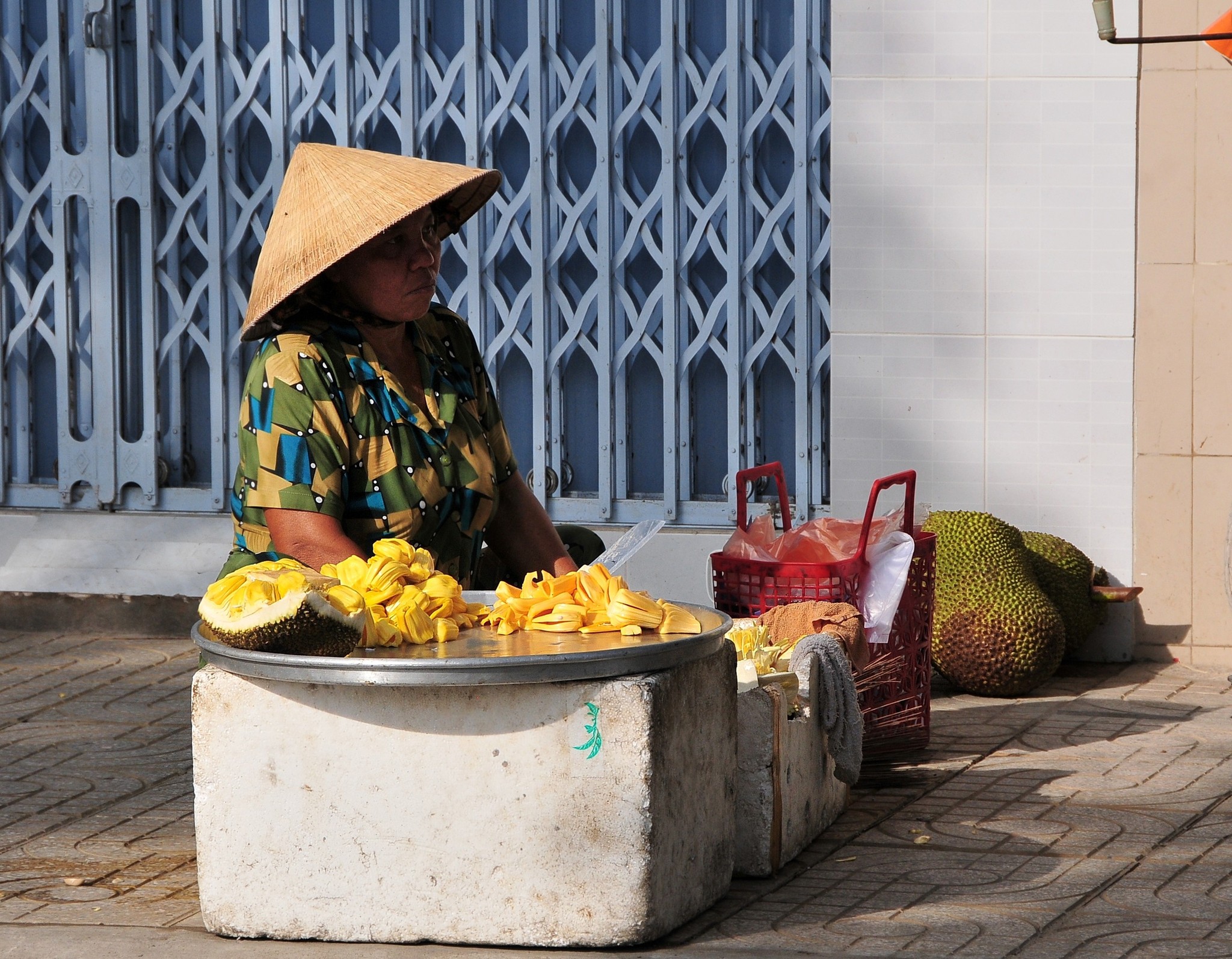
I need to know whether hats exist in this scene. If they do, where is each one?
[240,142,503,344]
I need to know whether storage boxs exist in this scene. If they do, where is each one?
[734,619,858,883]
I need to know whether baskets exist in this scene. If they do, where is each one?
[709,461,937,760]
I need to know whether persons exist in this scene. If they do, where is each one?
[207,140,609,592]
